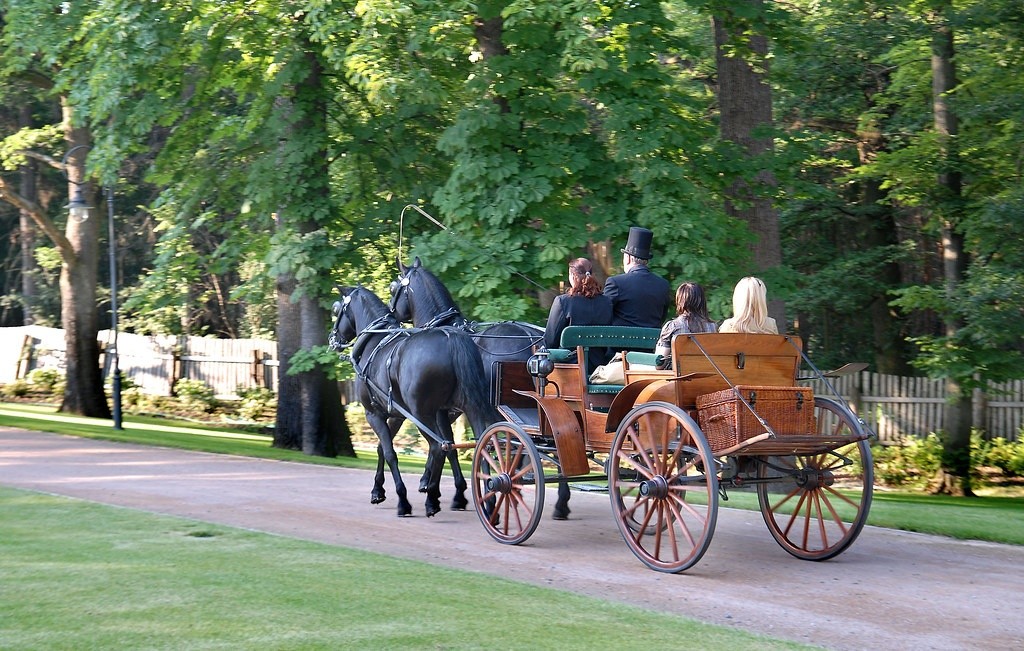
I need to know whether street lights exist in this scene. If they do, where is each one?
[61,132,128,432]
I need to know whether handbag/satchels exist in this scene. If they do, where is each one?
[588,352,629,385]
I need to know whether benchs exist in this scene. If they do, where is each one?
[535,326,661,394]
[622,332,802,407]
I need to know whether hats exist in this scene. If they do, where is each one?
[620,227,654,261]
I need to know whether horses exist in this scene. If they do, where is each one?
[328,257,575,518]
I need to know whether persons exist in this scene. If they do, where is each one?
[718,277,779,335]
[601,226,669,328]
[656,281,718,370]
[532,258,612,391]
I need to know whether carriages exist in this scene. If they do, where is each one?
[324,257,880,575]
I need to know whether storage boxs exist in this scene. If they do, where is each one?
[696,385,816,453]
